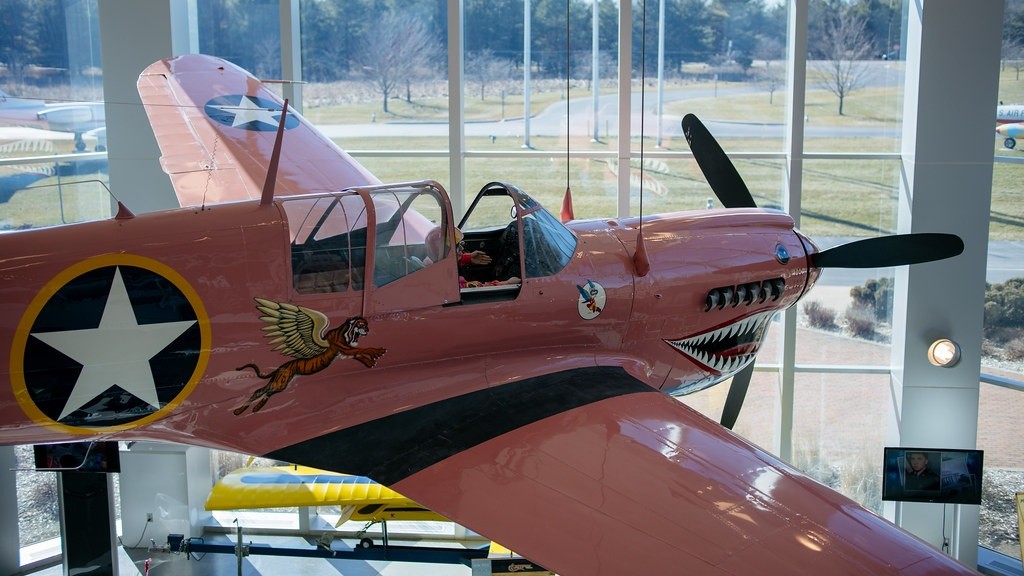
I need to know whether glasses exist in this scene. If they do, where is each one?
[456,241,465,253]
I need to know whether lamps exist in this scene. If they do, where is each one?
[928,337,962,368]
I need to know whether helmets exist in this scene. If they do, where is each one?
[425,225,464,263]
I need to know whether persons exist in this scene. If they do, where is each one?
[906,452,941,491]
[423,225,521,288]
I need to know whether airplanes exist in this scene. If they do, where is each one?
[0,90,107,153]
[0,54,986,576]
[994,104,1024,149]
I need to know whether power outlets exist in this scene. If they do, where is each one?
[146,513,154,522]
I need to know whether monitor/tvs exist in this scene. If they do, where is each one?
[881,445,985,507]
[33,442,121,475]
